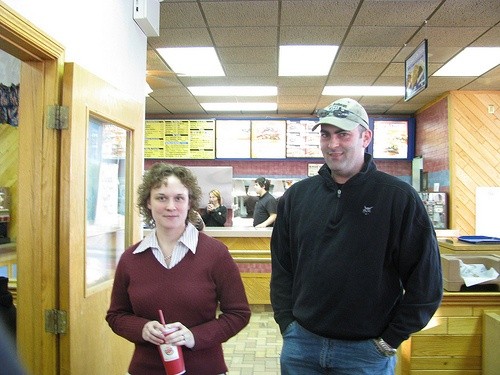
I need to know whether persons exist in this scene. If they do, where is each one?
[201,189,227,227]
[253,177,277,228]
[105,163,252,375]
[269,98,442,375]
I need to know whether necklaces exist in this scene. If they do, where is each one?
[165,256,171,260]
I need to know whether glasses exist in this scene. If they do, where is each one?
[318,109,367,124]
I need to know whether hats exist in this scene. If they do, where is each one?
[312,98,370,131]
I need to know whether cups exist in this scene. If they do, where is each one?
[433,182,440,192]
[207,203,214,211]
[157,328,188,375]
